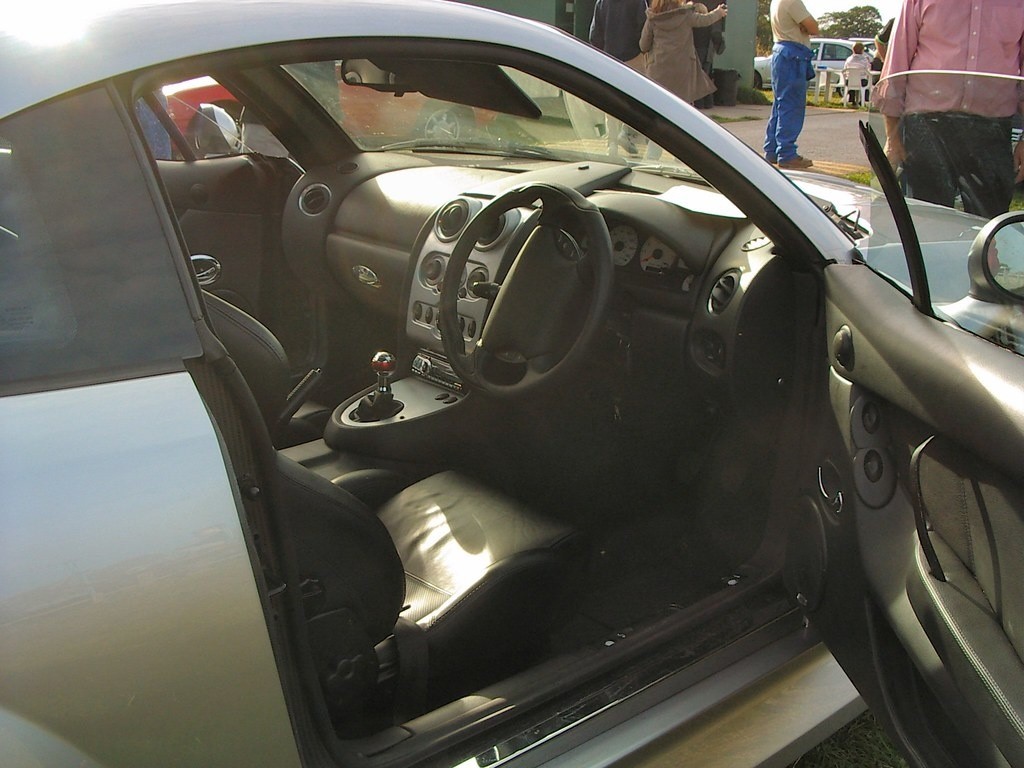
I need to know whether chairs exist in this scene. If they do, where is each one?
[201,288,591,741]
[841,67,872,108]
[12,104,331,450]
[819,67,844,102]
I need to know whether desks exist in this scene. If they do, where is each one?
[836,69,880,107]
[813,68,835,103]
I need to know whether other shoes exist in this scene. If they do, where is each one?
[617,134,638,154]
[777,156,813,168]
[606,154,628,164]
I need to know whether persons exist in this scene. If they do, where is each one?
[874,0,1024,221]
[587,0,727,164]
[235,60,346,160]
[874,16,896,65]
[764,0,819,169]
[843,42,871,107]
[870,48,884,87]
[136,83,172,161]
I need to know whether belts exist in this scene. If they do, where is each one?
[929,111,986,120]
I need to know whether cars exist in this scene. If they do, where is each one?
[752,37,873,90]
[1,0,1024,768]
